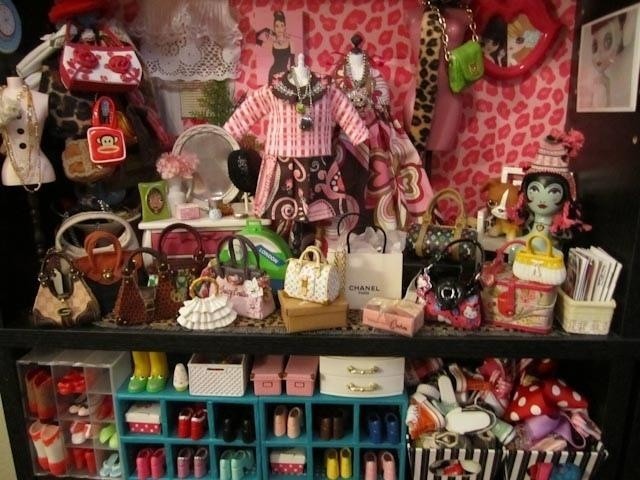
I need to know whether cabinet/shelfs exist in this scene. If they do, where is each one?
[137,200,277,288]
[0,0,640,478]
[253,391,411,480]
[14,342,136,479]
[114,370,266,479]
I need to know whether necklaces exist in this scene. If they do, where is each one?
[0,85,42,193]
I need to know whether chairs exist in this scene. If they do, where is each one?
[475,163,530,267]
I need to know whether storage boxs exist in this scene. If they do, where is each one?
[361,295,426,341]
[476,259,557,340]
[406,436,505,480]
[122,402,164,437]
[275,287,351,334]
[506,435,614,480]
[282,354,322,399]
[185,348,249,399]
[249,355,288,398]
[315,352,406,400]
[268,450,306,477]
[553,286,619,338]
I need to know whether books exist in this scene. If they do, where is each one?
[564,246,623,301]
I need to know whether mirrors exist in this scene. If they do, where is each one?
[169,124,244,207]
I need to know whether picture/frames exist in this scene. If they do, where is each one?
[574,0,640,118]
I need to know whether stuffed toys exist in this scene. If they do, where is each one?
[478,179,525,243]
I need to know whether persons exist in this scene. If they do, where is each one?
[509,170,571,257]
[222,54,372,223]
[326,30,438,229]
[256,10,296,83]
[0,75,57,189]
